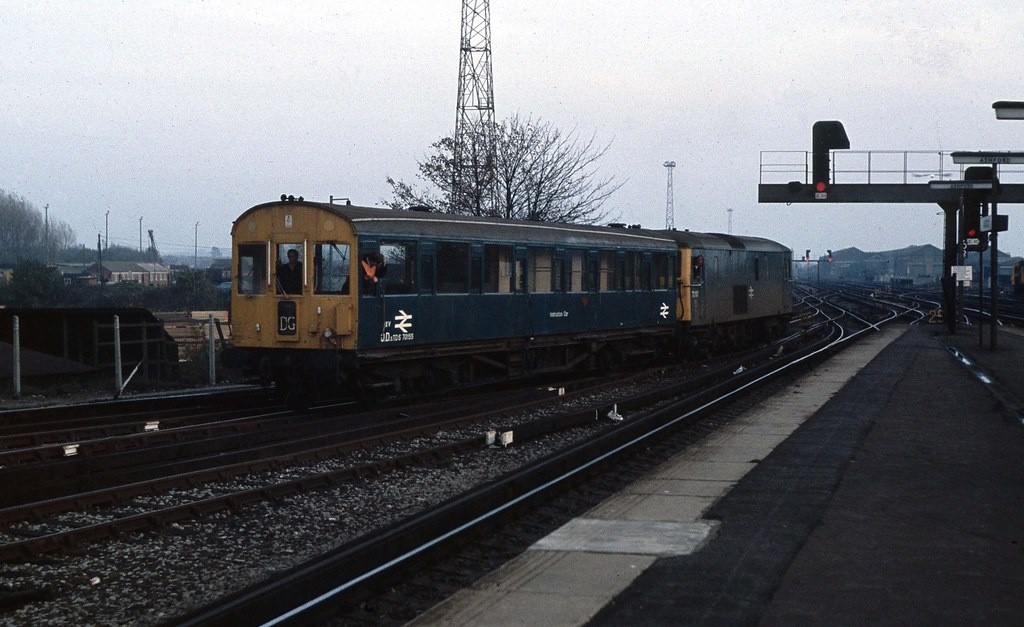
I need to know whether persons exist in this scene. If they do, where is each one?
[277,249,302,296]
[339,251,387,297]
[693,254,704,280]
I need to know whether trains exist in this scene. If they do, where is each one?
[0,308,178,392]
[228,193,797,407]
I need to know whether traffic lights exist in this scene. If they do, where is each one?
[960,202,979,249]
[828,251,832,261]
[805,251,810,261]
[816,156,827,192]
